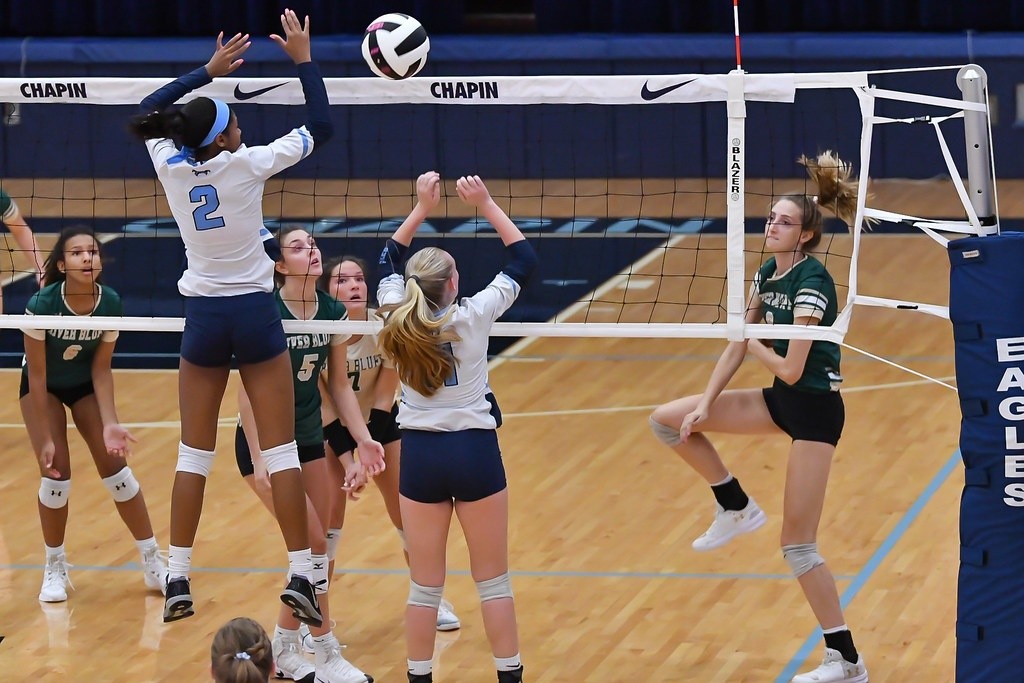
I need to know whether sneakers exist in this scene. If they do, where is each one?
[436,598,459,630]
[280,574,327,627]
[39,552,75,602]
[40,602,71,627]
[792,647,868,683]
[314,637,373,683]
[298,622,316,654]
[271,636,315,683]
[144,598,181,631]
[692,496,766,551]
[141,543,169,597]
[163,573,194,622]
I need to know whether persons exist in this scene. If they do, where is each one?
[211,617,274,682]
[1,180,45,315]
[648,152,869,682]
[318,256,461,631]
[375,172,544,683]
[233,223,385,682]
[136,10,333,624]
[18,226,174,604]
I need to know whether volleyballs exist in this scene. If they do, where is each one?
[360,13,430,81]
